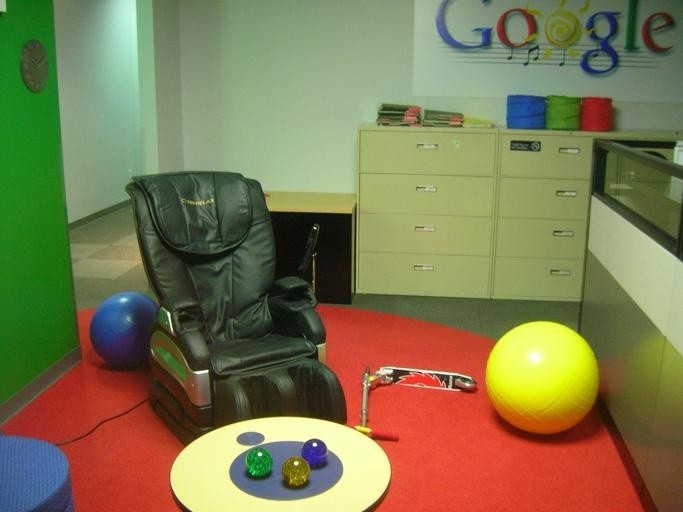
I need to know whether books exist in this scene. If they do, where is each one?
[374,103,494,127]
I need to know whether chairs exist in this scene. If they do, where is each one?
[124,171,348,446]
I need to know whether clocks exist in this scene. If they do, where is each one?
[20,40,48,93]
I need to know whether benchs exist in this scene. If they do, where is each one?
[263,192,358,307]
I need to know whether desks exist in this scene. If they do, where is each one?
[169,414,390,512]
